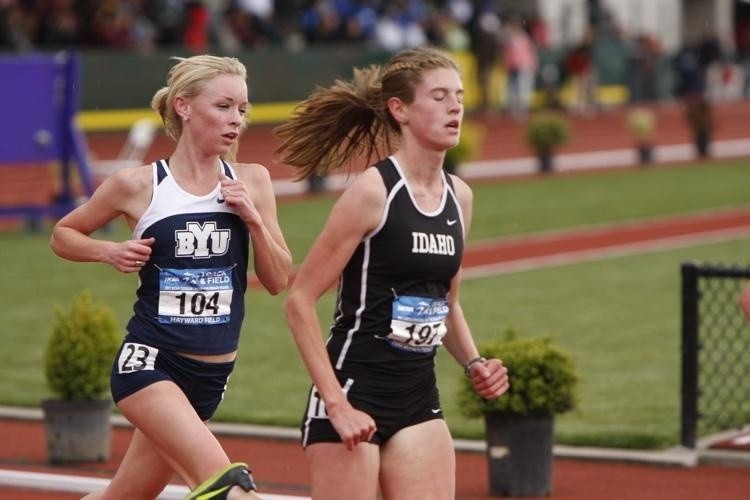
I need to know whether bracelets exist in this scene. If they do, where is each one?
[464,357,488,377]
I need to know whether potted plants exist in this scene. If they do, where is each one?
[451,327,586,498]
[39,288,125,466]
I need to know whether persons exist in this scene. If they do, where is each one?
[50,53,293,500]
[271,45,510,499]
[526,8,750,120]
[1,1,541,122]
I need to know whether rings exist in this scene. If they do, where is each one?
[135,261,141,266]
[224,189,233,197]
